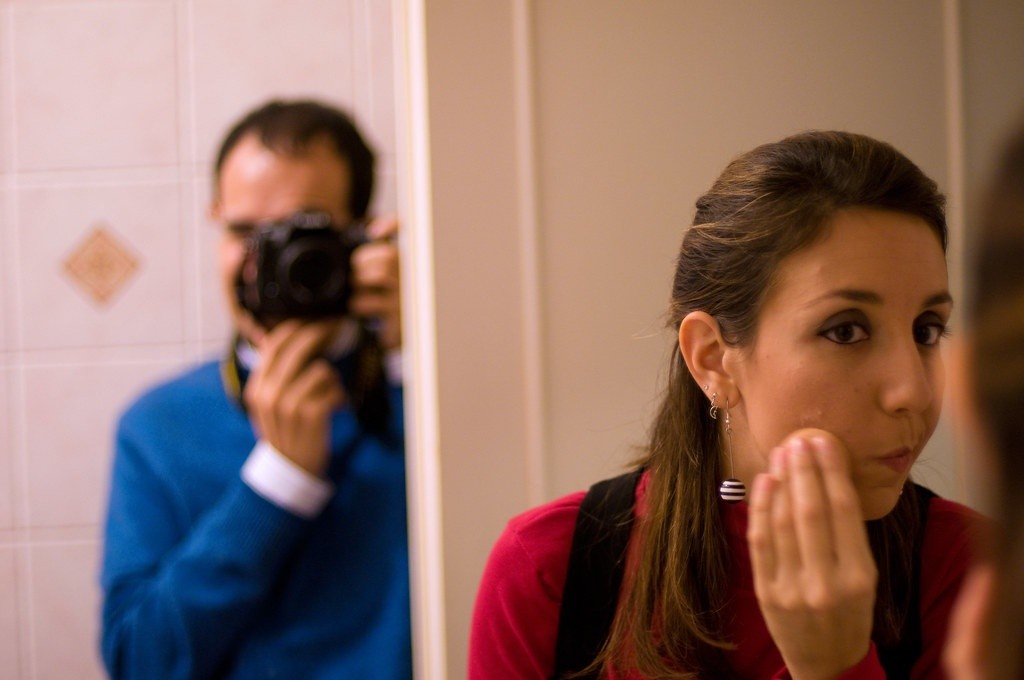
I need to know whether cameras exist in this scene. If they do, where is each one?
[251,210,372,324]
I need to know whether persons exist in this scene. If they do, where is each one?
[97,99,413,680]
[467,131,1001,680]
